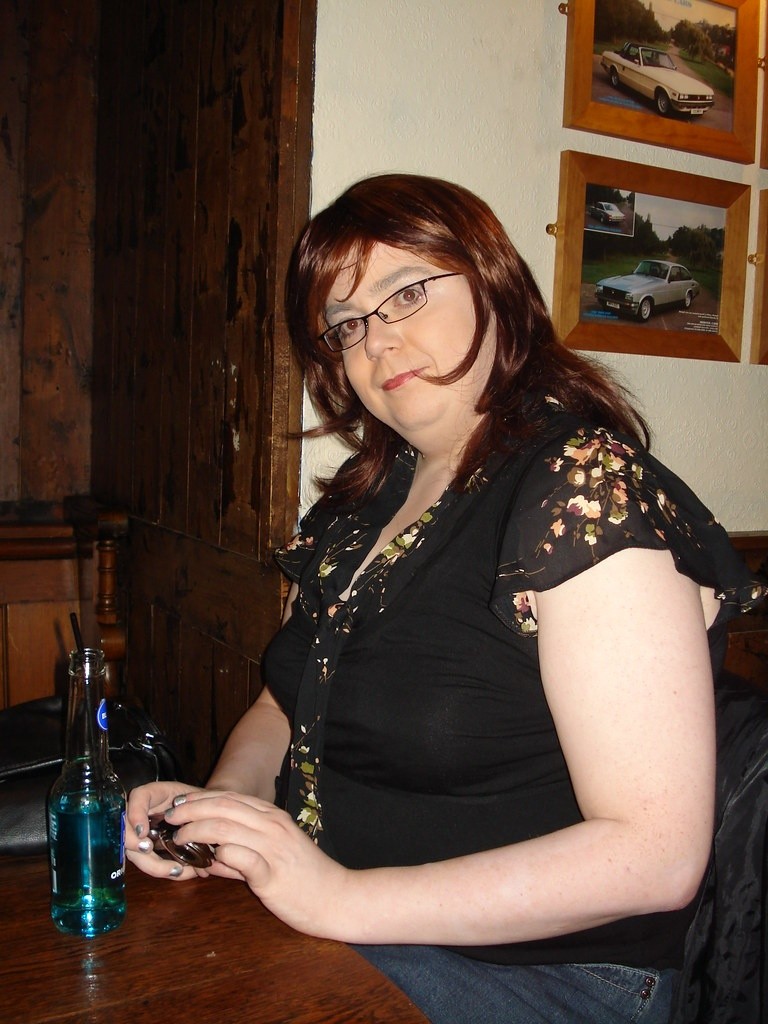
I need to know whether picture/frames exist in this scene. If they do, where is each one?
[558,1,762,164]
[748,189,768,365]
[544,150,751,362]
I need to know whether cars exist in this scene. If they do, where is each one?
[594,260,701,321]
[599,42,716,118]
[588,202,626,224]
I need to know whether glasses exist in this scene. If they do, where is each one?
[317,271,461,353]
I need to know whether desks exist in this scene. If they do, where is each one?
[1,850,433,1024]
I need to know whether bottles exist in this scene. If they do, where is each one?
[46,648,129,939]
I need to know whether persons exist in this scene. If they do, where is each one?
[122,174,768,1024]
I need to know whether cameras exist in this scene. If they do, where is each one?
[149,811,221,868]
